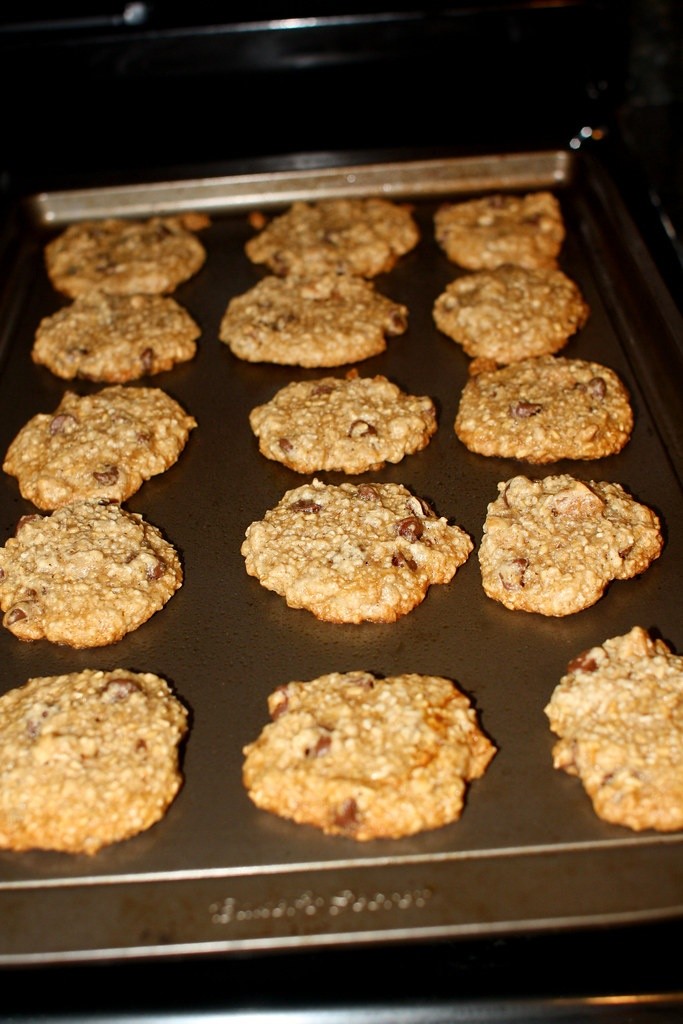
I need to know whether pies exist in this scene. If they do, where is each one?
[1,191,683,857]
